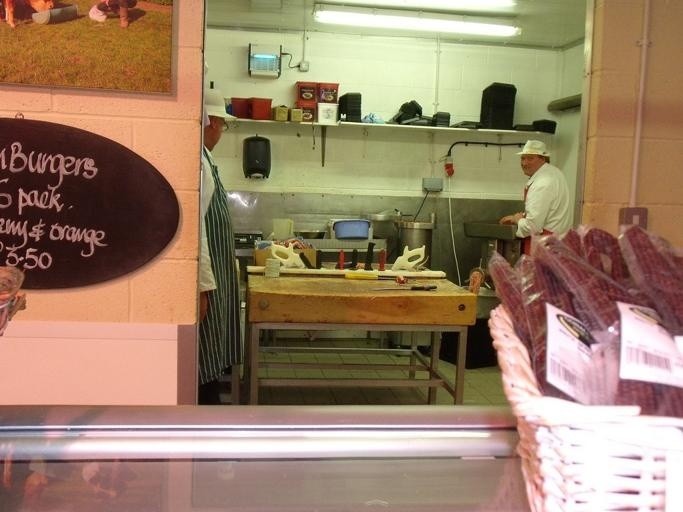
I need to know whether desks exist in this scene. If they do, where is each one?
[244,261,478,406]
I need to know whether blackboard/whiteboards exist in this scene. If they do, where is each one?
[0,117,181,291]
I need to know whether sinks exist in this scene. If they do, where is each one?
[463,220,520,241]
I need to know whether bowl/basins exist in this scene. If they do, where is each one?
[294,231,326,239]
[463,285,501,321]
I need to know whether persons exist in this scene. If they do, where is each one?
[199,88,244,405]
[500,139,574,256]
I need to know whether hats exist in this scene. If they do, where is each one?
[516,139,551,157]
[205,89,238,119]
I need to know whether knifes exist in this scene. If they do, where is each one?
[368,284,437,292]
[300,243,385,272]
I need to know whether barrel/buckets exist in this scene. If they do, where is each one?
[317,102,340,124]
[396,219,436,270]
[261,218,293,241]
[231,96,250,119]
[249,97,273,121]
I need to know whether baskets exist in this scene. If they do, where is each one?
[488,304,683,512]
